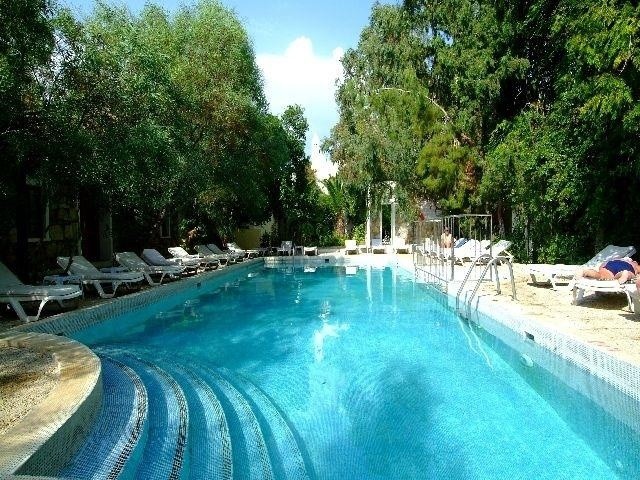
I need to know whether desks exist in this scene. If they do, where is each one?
[44,275,81,309]
[101,267,129,293]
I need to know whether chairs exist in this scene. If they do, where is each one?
[44,255,144,299]
[113,242,239,286]
[343,233,517,269]
[0,263,82,322]
[239,241,318,261]
[526,243,640,318]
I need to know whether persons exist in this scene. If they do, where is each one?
[559,256,640,288]
[440,227,456,248]
